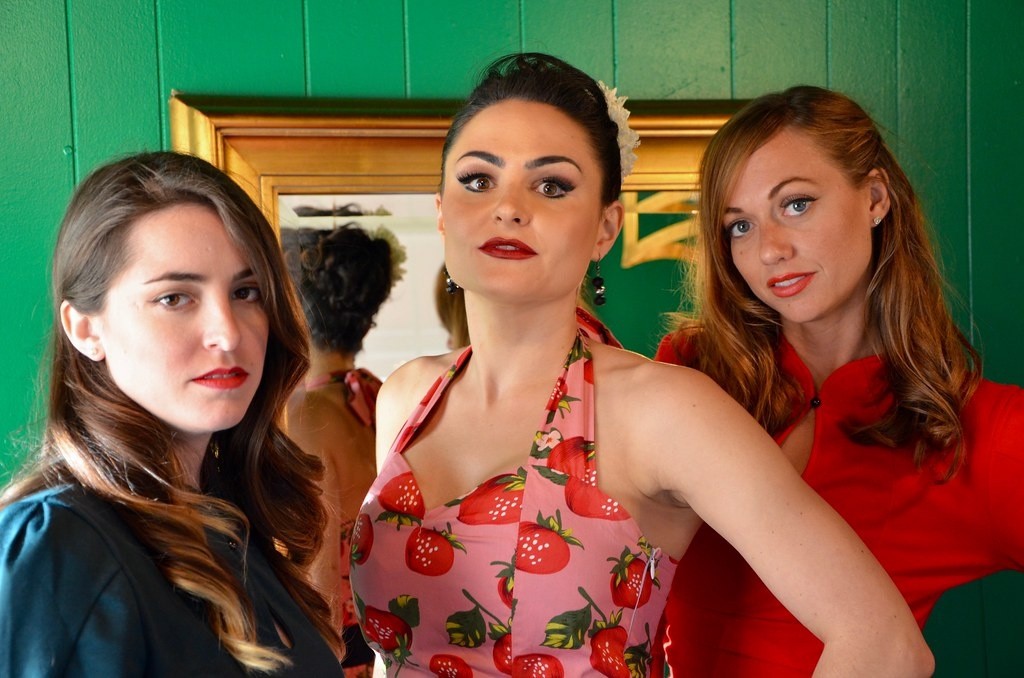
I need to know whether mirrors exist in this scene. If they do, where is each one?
[168,93,753,678]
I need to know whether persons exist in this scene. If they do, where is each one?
[0,150,347,678]
[280,206,406,678]
[434,263,471,351]
[655,85,1024,678]
[349,54,935,678]
